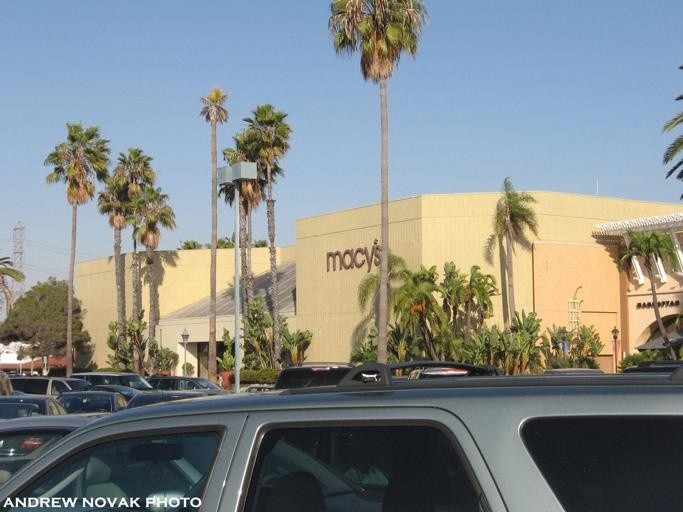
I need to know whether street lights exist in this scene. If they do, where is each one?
[610,326,619,374]
[180,326,190,377]
[216,162,257,394]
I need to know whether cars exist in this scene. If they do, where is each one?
[0,362,498,490]
[542,369,605,375]
[0,359,683,512]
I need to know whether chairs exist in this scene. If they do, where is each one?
[250,469,327,512]
[71,448,131,507]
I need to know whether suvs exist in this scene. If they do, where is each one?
[623,359,682,374]
